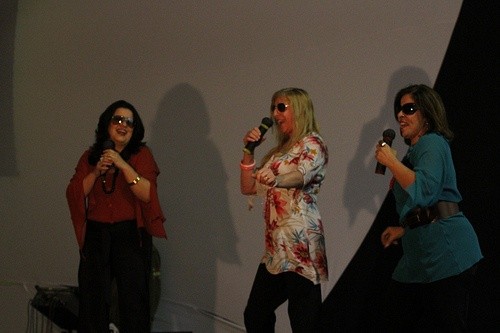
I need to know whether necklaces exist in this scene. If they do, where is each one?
[102,168,119,194]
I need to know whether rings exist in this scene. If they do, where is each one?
[382,142,386,146]
[100,157,103,162]
[265,176,269,180]
[261,173,263,176]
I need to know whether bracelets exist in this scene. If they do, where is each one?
[128,175,141,187]
[240,159,256,171]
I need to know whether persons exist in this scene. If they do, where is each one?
[66,100,167,333]
[239,87,329,333]
[374,84,485,333]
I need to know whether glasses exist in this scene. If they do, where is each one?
[396,103,418,115]
[111,114,135,128]
[271,103,289,112]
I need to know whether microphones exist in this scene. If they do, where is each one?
[101,140,114,175]
[375,129,396,175]
[243,117,273,155]
